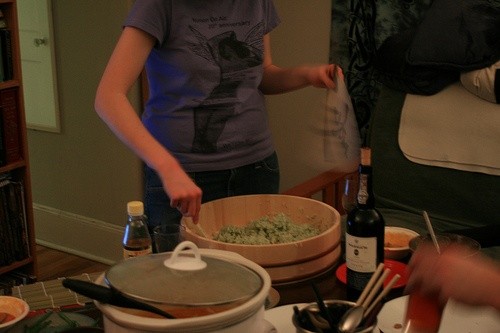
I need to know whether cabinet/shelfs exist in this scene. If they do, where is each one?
[0,0,39,297]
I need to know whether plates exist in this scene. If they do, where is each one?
[377,295,500,333]
[337,259,409,289]
[264,303,307,333]
[0,296,30,328]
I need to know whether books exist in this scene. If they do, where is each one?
[0,174,38,295]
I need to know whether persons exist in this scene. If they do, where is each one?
[373,0,500,96]
[405,240,500,308]
[94,0,341,255]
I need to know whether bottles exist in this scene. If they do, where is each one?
[346,147,384,299]
[122,201,152,260]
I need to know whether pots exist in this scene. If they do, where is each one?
[92,241,271,333]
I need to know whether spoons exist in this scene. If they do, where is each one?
[338,269,391,332]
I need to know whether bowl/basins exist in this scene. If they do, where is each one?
[385,226,420,259]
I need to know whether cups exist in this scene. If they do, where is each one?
[409,233,481,257]
[154,223,186,253]
[291,300,378,333]
